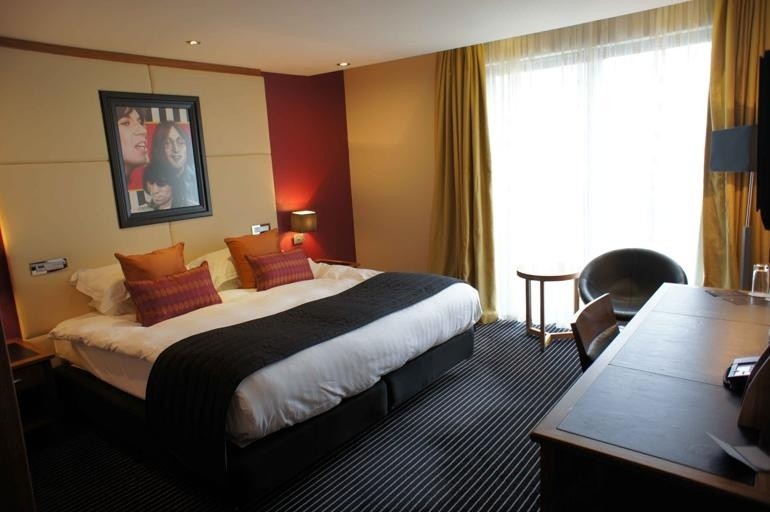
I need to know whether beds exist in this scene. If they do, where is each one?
[46,258,483,504]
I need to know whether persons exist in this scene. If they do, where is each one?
[116,106,151,180]
[148,121,198,201]
[136,160,196,211]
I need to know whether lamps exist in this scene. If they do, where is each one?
[710,122,762,290]
[290,209,318,245]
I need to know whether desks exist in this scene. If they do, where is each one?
[530,280,770,510]
[516,262,583,353]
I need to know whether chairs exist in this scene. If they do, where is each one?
[570,291,620,372]
[579,248,689,322]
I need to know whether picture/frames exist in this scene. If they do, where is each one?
[98,90,212,230]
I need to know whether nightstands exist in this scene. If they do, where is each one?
[315,258,361,269]
[6,336,55,433]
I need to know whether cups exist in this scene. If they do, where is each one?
[751,263,769,296]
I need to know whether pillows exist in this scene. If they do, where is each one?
[68,228,314,327]
